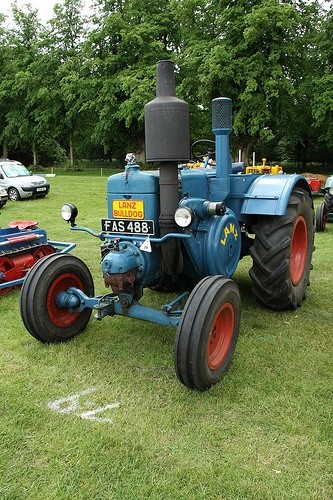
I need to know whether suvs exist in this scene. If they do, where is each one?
[0,158,52,203]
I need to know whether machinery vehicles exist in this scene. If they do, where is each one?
[186,147,333,232]
[17,59,317,393]
